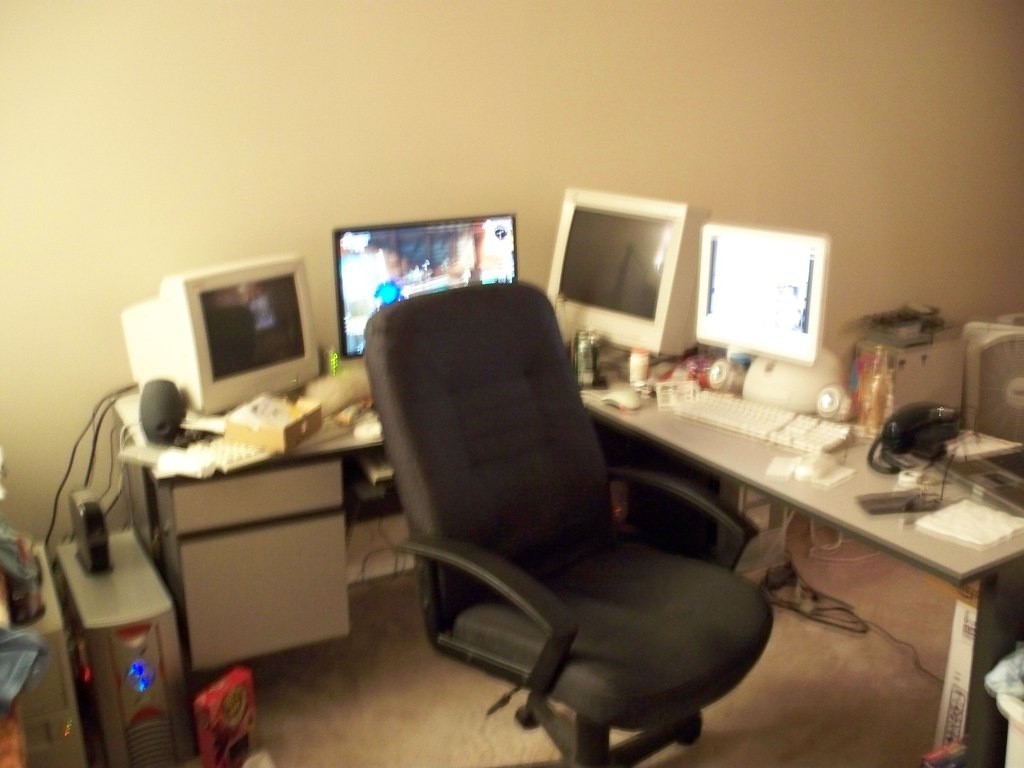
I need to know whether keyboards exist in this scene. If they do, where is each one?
[190,414,348,473]
[678,390,854,454]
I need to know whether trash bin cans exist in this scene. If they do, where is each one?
[996,648,1024,768]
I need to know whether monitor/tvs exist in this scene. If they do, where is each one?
[332,211,518,361]
[544,188,710,360]
[694,221,845,414]
[119,256,320,418]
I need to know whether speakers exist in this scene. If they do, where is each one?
[140,381,185,445]
[816,386,855,422]
[708,362,745,393]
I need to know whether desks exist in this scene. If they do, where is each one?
[109,342,1024,759]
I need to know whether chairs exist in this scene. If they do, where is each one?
[364,283,773,768]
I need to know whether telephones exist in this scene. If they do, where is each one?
[882,402,961,452]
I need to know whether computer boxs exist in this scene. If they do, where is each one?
[53,529,200,768]
[0,544,86,768]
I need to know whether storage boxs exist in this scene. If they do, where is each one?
[920,740,968,768]
[193,664,256,768]
[223,392,324,455]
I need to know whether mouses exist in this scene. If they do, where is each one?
[602,387,642,410]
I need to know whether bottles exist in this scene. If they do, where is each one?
[629,348,649,385]
[863,343,890,437]
[552,292,572,360]
